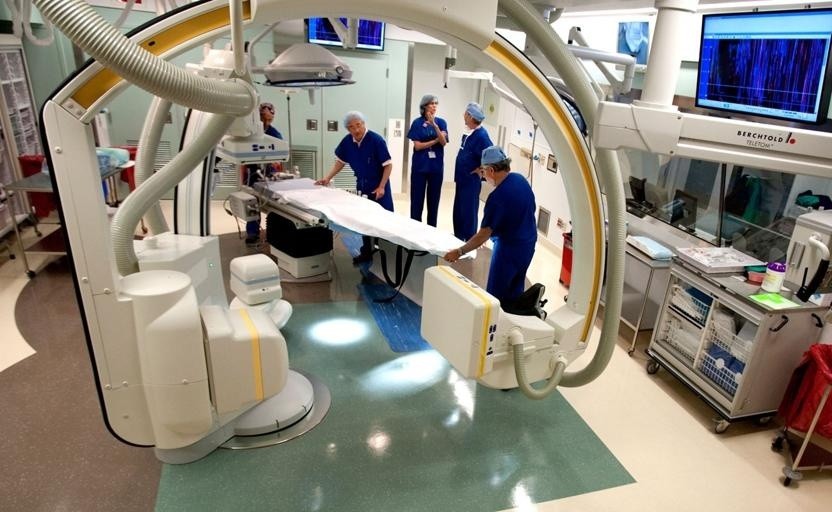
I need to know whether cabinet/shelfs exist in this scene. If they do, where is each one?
[0,33,46,241]
[645,254,830,434]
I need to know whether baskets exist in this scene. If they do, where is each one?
[670,284,710,325]
[663,317,702,360]
[699,349,742,395]
[707,320,752,364]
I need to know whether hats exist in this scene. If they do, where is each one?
[480,145,506,165]
[466,102,485,121]
[259,102,275,114]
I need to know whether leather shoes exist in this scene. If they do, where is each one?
[245,235,256,244]
[353,254,373,263]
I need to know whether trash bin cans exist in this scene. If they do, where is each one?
[775,342,832,439]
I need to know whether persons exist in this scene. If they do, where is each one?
[239,101,286,249]
[443,144,547,316]
[404,94,451,228]
[452,101,492,254]
[313,111,394,266]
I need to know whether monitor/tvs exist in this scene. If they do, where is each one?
[695,8,832,126]
[304,17,386,51]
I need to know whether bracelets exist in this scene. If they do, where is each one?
[458,247,465,257]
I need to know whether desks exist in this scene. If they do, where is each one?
[563,231,680,357]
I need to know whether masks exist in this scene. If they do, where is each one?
[459,126,476,149]
